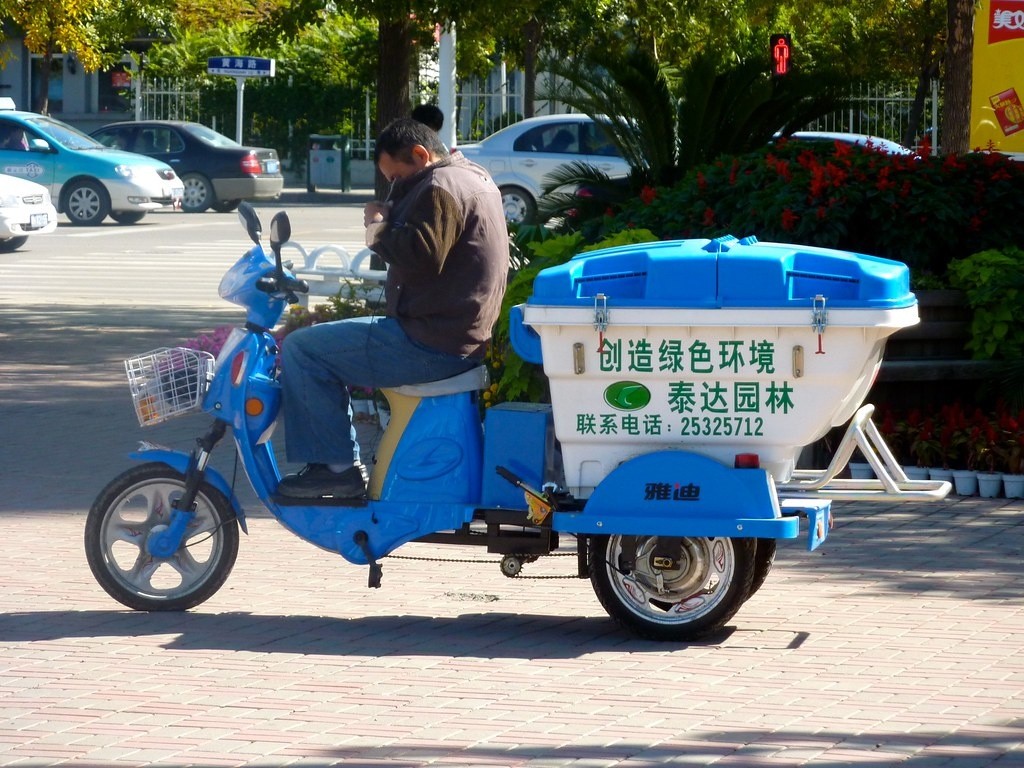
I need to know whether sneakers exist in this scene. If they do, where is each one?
[276,463,367,499]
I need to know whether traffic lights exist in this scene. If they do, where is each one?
[770,31,792,76]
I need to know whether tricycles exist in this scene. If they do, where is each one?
[85,201,953,642]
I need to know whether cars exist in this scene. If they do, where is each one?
[87,121,284,214]
[770,130,920,161]
[449,113,680,230]
[954,149,1024,167]
[0,96,186,225]
[0,174,58,253]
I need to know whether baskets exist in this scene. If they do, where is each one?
[125,347,215,428]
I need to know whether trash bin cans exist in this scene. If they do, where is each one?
[510,227,924,506]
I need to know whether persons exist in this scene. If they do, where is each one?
[278,116,510,498]
[7,124,28,150]
[544,129,574,152]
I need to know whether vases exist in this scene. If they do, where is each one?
[928,467,955,482]
[976,469,1004,497]
[903,466,930,480]
[1003,475,1024,498]
[848,463,875,479]
[952,470,981,495]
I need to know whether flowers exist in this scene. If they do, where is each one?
[848,399,1023,474]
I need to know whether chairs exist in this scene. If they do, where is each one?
[140,130,157,154]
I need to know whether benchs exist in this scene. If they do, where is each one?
[266,241,390,308]
